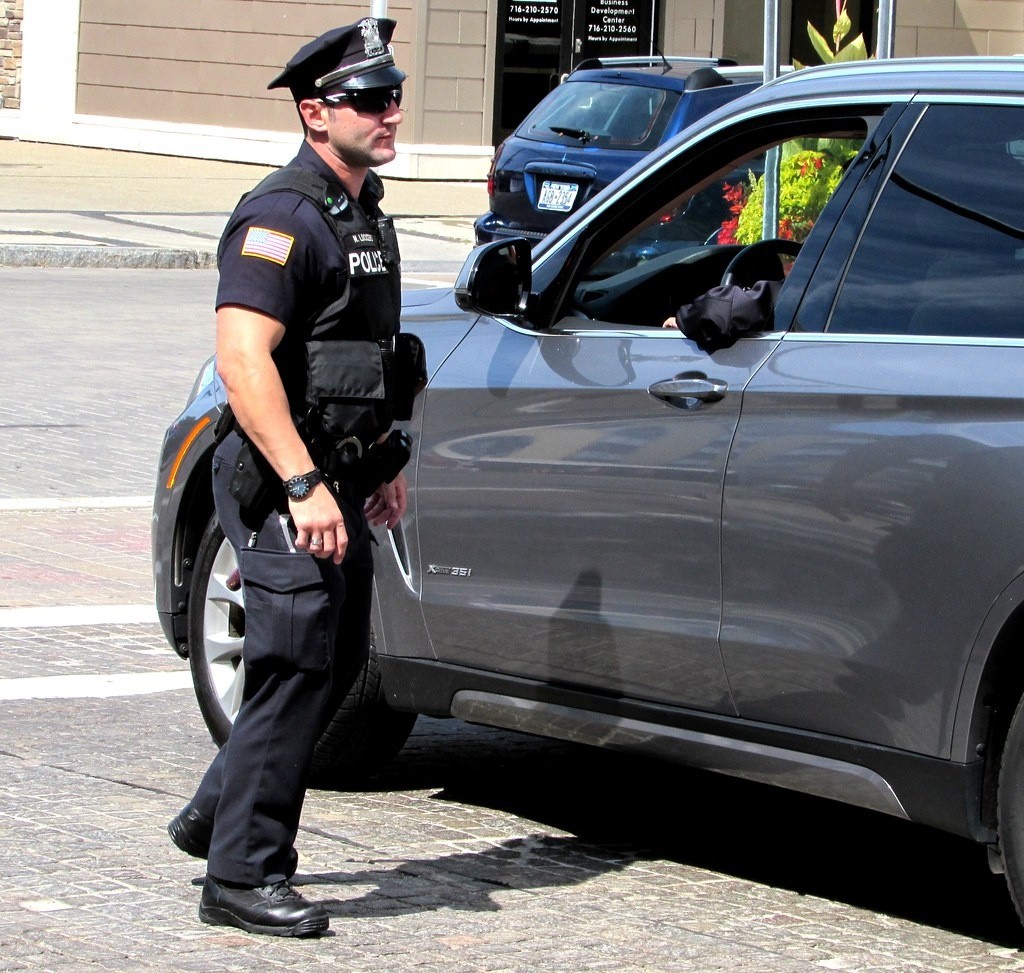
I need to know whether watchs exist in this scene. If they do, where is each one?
[281,466,323,500]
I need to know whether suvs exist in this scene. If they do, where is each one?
[471,56,815,277]
[150,54,1023,927]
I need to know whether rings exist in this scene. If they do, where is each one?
[311,538,323,546]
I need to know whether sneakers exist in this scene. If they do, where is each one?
[167,803,219,860]
[199,872,328,938]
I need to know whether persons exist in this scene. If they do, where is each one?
[166,15,408,940]
[663,280,781,352]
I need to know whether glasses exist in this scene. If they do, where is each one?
[319,83,404,115]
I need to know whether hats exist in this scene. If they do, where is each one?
[266,17,407,103]
